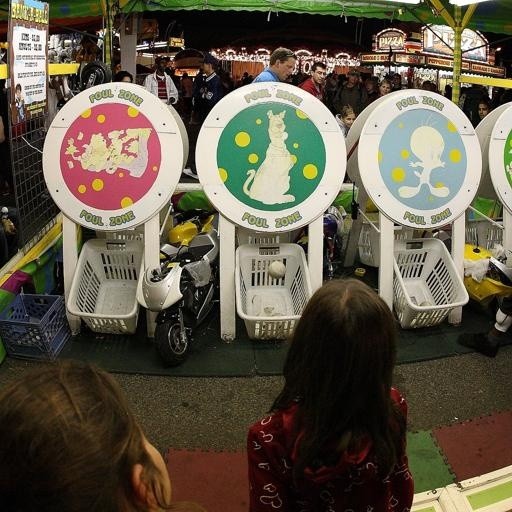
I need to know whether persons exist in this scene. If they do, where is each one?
[423,81,511,129]
[246,278,415,512]
[14,84,25,124]
[291,62,403,129]
[116,57,192,126]
[456,294,512,358]
[0,357,204,512]
[183,55,224,180]
[249,47,298,84]
[224,71,255,93]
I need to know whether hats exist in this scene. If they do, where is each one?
[198,58,214,63]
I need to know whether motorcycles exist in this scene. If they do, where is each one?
[142,208,221,363]
[319,196,349,282]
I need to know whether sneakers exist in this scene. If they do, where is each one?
[183,168,197,179]
[458,332,499,358]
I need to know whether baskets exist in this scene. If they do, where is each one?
[67,224,144,335]
[0,293,72,364]
[358,220,470,329]
[235,225,313,341]
[465,217,503,250]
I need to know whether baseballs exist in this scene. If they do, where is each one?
[419,300,433,314]
[268,261,286,278]
